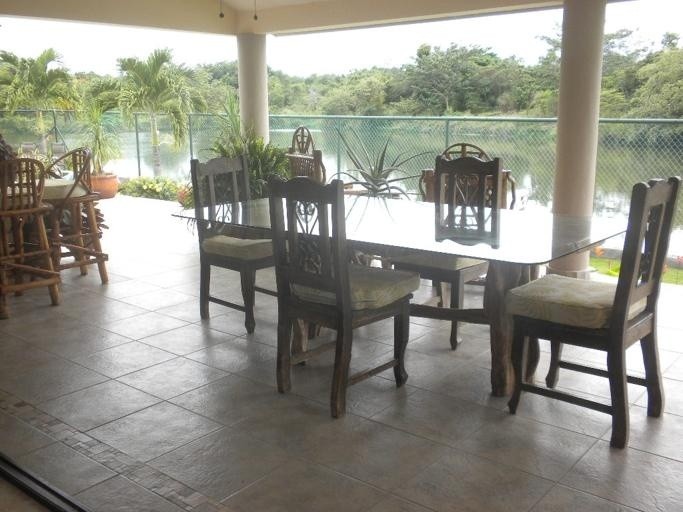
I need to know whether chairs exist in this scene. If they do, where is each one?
[0,146,108,320]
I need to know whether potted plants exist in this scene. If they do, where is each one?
[84,97,121,199]
